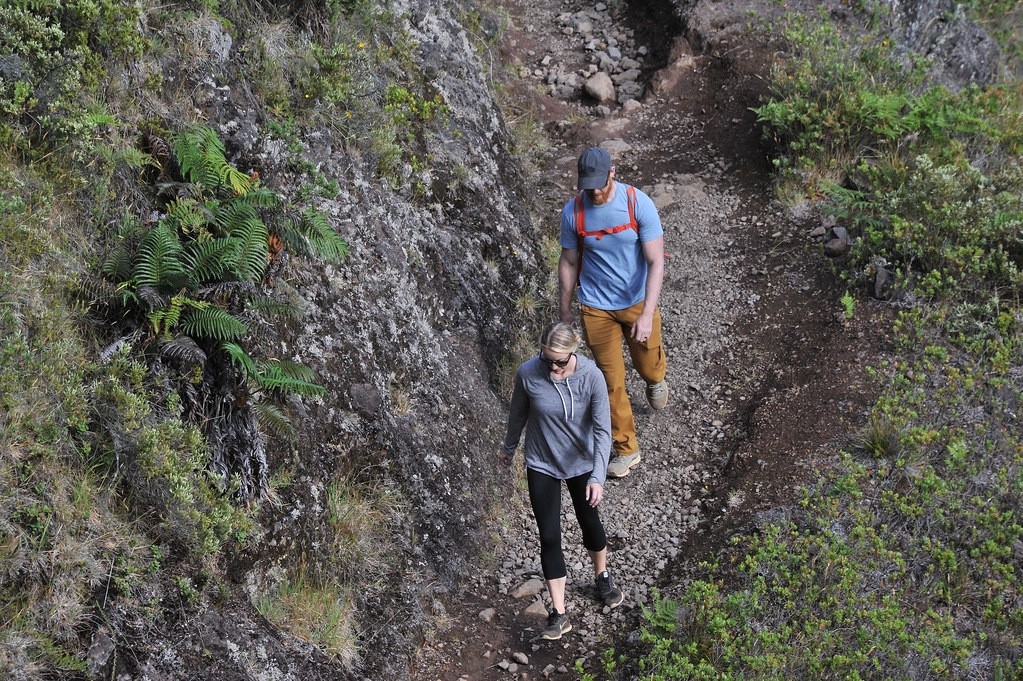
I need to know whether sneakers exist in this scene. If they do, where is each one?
[606,451,640,477]
[646,379,668,409]
[594,571,623,608]
[540,608,572,639]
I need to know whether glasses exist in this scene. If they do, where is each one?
[585,171,611,191]
[539,348,573,367]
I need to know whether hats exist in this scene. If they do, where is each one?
[577,147,611,189]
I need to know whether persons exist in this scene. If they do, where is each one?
[500,322,624,640]
[558,146,669,477]
